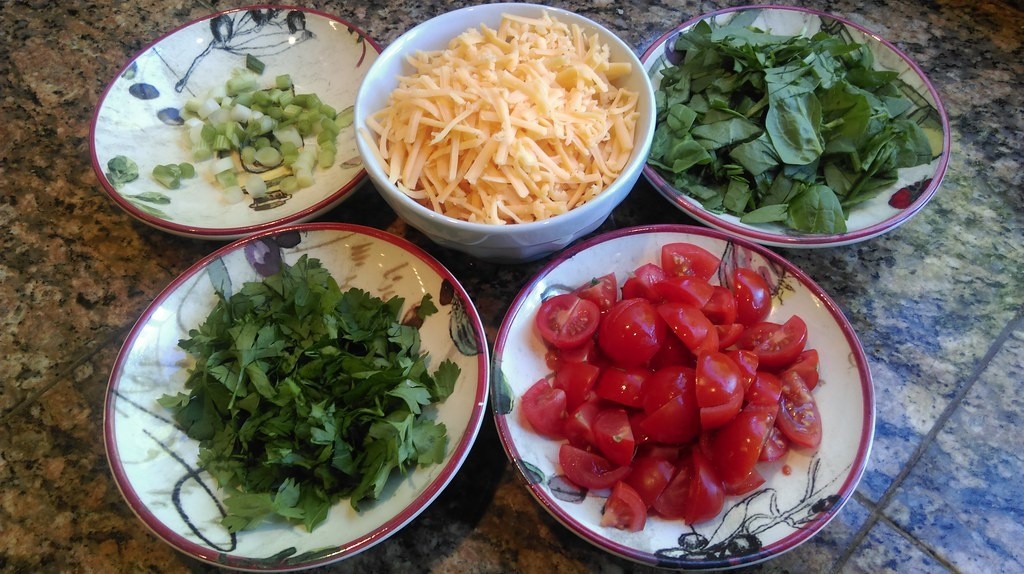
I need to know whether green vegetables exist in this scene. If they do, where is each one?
[156,253,461,536]
[645,18,933,236]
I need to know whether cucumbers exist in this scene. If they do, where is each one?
[152,50,340,199]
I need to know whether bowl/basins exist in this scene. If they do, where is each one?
[355,4,657,265]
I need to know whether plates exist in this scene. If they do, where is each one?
[492,224,876,570]
[636,5,951,247]
[89,6,381,238]
[104,222,491,574]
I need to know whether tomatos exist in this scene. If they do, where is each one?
[519,242,822,532]
